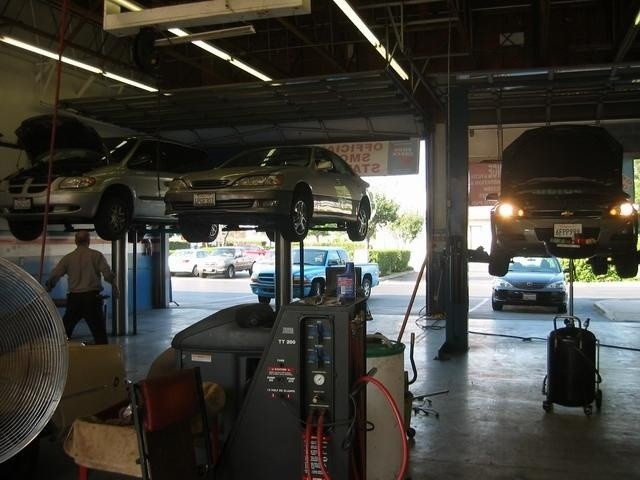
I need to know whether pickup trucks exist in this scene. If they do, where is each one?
[250,248,380,304]
[196,248,257,278]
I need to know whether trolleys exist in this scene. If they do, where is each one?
[544,316,601,415]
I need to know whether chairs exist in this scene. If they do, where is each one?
[130,365,213,480]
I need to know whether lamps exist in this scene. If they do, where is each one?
[114,1,280,86]
[1,35,172,96]
[334,0,408,80]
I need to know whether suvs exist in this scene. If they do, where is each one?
[488,125,640,276]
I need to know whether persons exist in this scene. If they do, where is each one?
[46,230,121,348]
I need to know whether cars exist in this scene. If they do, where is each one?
[491,254,566,312]
[1,116,220,243]
[168,249,210,276]
[165,145,377,244]
